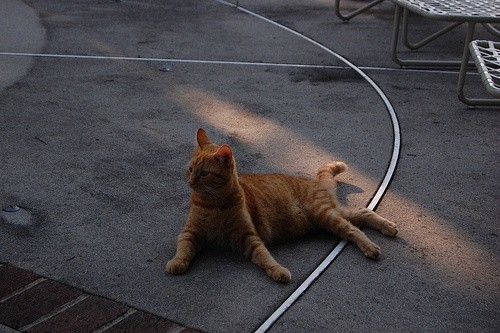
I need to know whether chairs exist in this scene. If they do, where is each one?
[333,0,500,110]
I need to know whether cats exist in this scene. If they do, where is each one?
[165,128,400,283]
